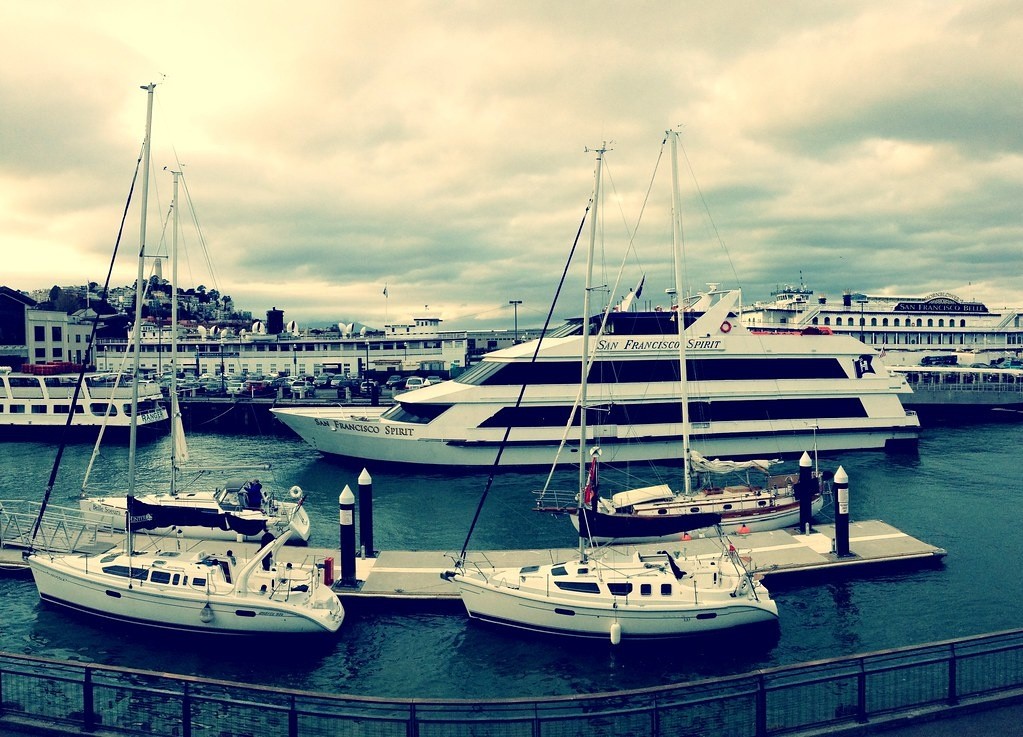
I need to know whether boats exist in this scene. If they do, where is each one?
[271,287,922,467]
[1,374,170,435]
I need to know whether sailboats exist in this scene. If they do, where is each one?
[25,84,349,639]
[75,165,315,545]
[438,139,781,648]
[527,128,831,541]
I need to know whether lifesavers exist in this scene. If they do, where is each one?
[720,320,732,333]
[738,577,750,595]
[290,486,302,498]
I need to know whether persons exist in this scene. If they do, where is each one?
[255,527,275,571]
[243,479,262,511]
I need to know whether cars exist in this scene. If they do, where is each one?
[92,372,442,398]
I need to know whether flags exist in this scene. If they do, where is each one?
[879,348,886,357]
[383,287,388,298]
